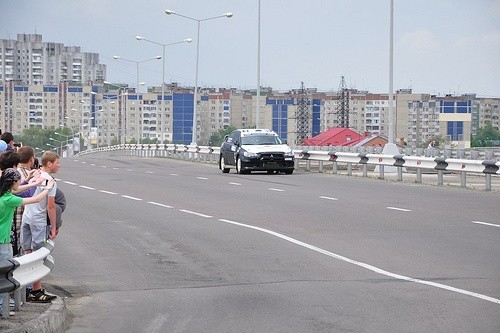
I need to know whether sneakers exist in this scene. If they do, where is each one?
[31,288,57,303]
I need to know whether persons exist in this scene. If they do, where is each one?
[428,140,436,148]
[20,151,60,301]
[0,168,55,315]
[0,131,39,196]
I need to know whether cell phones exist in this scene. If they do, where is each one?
[14,143,21,147]
[46,179,48,185]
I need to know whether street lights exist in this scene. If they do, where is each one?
[34,90,129,158]
[113,56,162,145]
[103,81,146,144]
[136,36,193,147]
[165,10,233,146]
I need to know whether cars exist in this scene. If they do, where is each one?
[219,128,295,175]
